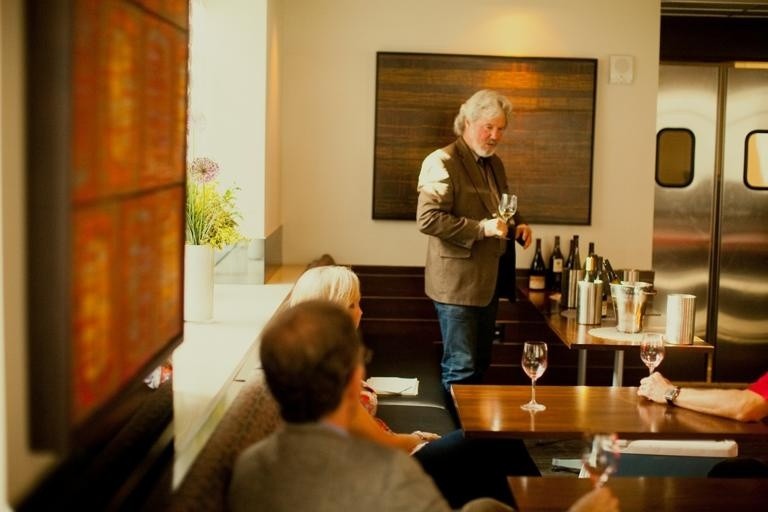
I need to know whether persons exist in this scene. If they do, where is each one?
[636,370,768,422]
[417,89,533,412]
[289,265,545,455]
[227,301,542,512]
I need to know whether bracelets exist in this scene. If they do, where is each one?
[411,430,432,445]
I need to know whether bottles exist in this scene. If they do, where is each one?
[569,235,581,270]
[596,260,608,317]
[582,256,595,283]
[528,239,547,291]
[566,241,574,268]
[582,242,595,281]
[603,259,621,285]
[547,236,564,291]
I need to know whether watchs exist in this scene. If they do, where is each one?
[661,385,682,404]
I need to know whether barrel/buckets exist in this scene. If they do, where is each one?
[608,279,659,335]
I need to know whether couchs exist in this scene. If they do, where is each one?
[168,337,452,512]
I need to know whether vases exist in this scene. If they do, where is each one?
[184,244,214,320]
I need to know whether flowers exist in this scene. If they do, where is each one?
[186,155,250,251]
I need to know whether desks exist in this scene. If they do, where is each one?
[451,381,768,477]
[506,477,768,512]
[514,270,715,387]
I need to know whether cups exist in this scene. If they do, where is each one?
[494,193,518,240]
[640,332,665,400]
[520,340,548,412]
